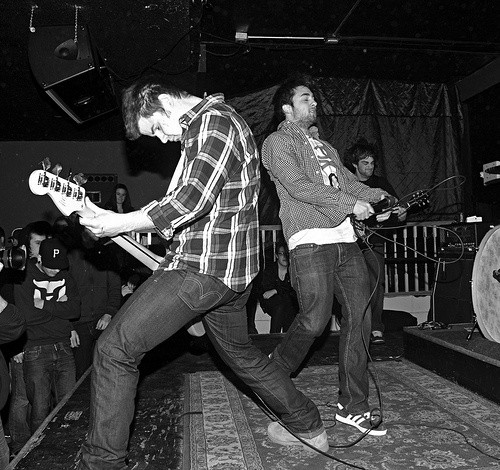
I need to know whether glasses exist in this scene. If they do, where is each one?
[278,252,288,256]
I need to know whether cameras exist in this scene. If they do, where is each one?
[0,246,25,270]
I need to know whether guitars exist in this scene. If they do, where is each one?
[356,189,431,251]
[28,157,207,337]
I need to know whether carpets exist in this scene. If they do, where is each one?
[180,357,500,470]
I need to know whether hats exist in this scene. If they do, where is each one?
[39,238,70,269]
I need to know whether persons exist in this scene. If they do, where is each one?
[259,82,398,437]
[344,139,406,343]
[104,183,136,214]
[258,243,297,334]
[76,80,328,469]
[0,211,120,470]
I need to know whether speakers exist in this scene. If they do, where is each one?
[428,257,475,322]
[28,23,121,124]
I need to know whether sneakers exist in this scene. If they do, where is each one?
[268,421,329,454]
[335,403,387,436]
[371,330,385,345]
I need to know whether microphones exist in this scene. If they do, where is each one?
[372,196,395,212]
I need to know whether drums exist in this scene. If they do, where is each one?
[470,223,500,343]
